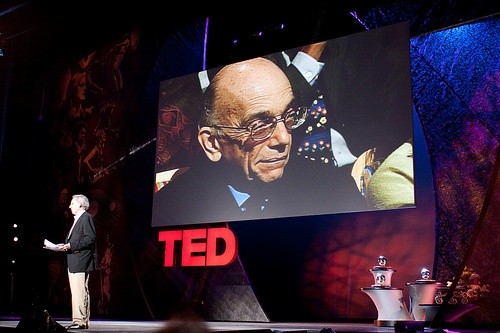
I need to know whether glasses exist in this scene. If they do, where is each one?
[207,105,309,139]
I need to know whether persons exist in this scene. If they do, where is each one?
[267,42,415,209]
[51,33,139,314]
[152,57,366,224]
[54,195,97,330]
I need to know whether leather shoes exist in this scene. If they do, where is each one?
[68,323,88,329]
